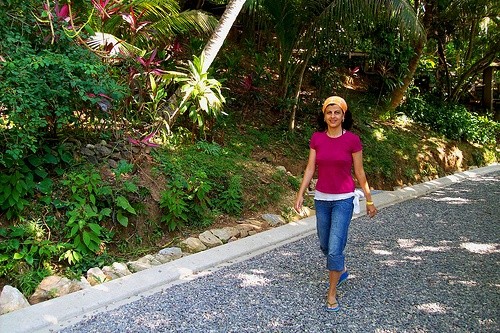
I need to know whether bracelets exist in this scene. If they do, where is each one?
[366,200,373,206]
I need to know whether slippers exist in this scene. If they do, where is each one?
[326,272,349,287]
[327,294,340,312]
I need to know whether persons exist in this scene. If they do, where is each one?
[293,96,379,312]
